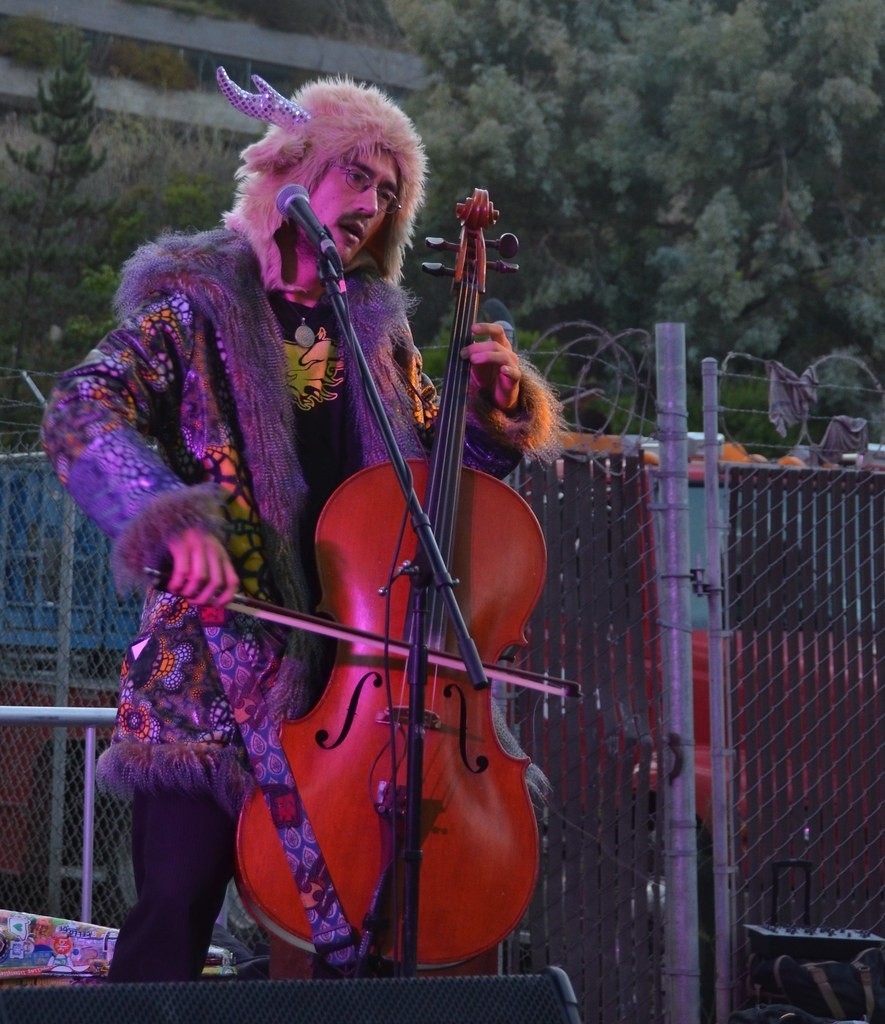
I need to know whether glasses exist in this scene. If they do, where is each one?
[335,162,402,215]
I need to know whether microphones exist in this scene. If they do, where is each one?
[278,186,346,270]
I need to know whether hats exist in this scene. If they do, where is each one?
[216,65,426,290]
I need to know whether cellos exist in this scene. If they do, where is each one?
[238,188,548,975]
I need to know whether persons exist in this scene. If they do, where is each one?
[39,65,561,983]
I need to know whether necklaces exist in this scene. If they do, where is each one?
[278,292,323,349]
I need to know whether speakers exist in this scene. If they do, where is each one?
[0,965,580,1023]
